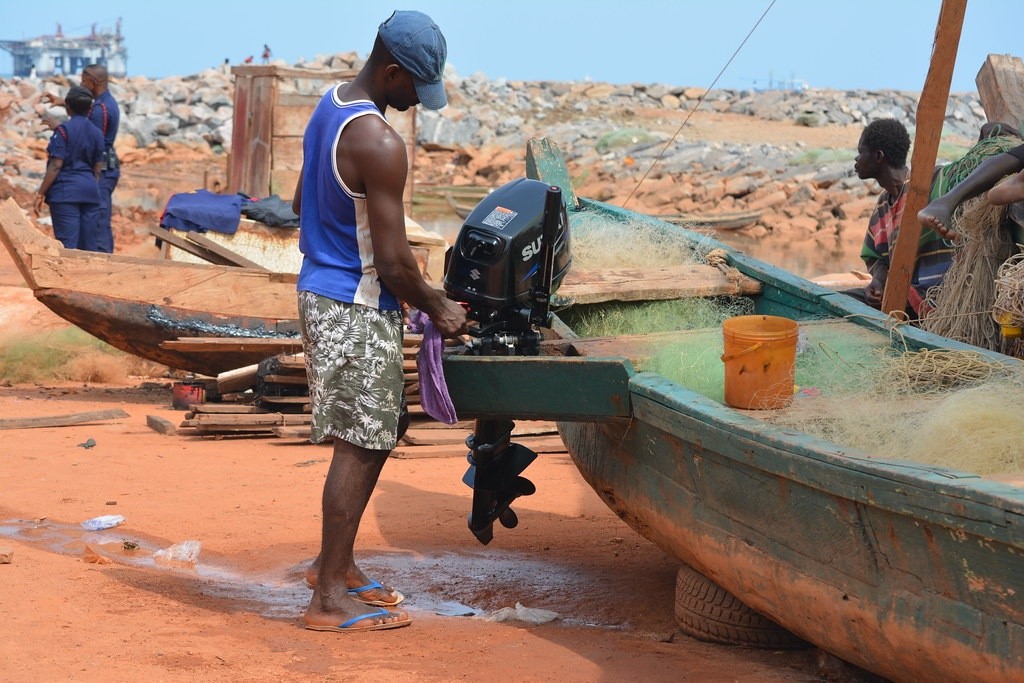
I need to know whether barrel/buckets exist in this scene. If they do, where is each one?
[172,381,204,409]
[719,314,801,410]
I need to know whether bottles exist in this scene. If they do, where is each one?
[81,515,126,531]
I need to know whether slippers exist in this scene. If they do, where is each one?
[304,607,412,632]
[303,577,404,607]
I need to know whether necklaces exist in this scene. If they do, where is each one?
[887,171,908,207]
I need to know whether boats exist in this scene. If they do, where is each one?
[0,189,457,386]
[640,208,764,232]
[446,136,1024,683]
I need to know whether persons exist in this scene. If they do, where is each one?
[81,65,120,254]
[291,9,468,630]
[918,144,1024,240]
[854,118,955,328]
[33,86,105,253]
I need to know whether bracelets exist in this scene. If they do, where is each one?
[37,193,43,199]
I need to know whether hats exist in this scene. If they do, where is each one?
[378,9,448,110]
[70,86,93,97]
